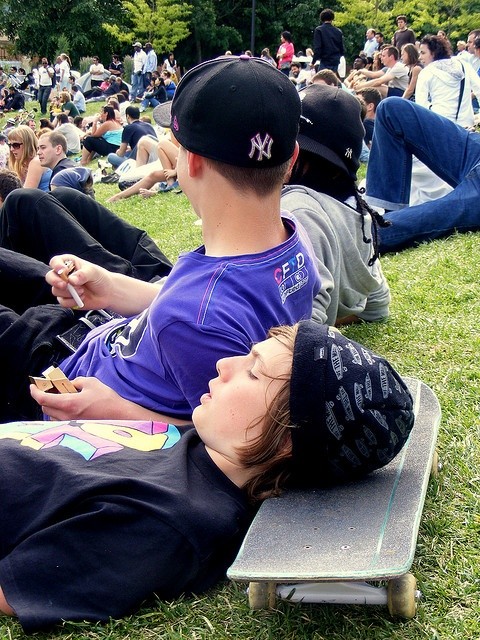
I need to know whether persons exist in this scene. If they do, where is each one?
[1,83,392,326]
[222,9,348,88]
[1,37,178,204]
[0,54,323,428]
[352,87,382,151]
[1,321,416,633]
[381,14,480,132]
[364,96,480,256]
[346,28,382,88]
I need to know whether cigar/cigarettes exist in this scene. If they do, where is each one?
[58,270,84,308]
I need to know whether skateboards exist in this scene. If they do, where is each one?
[225,376,443,618]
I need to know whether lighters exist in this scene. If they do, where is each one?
[63,261,85,296]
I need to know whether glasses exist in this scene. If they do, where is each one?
[7,143,23,149]
[42,62,47,64]
[94,60,96,61]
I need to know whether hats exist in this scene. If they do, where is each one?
[294,83,363,179]
[143,43,152,47]
[152,54,301,166]
[289,320,414,492]
[132,42,142,48]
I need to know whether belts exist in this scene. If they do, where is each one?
[35,308,123,378]
